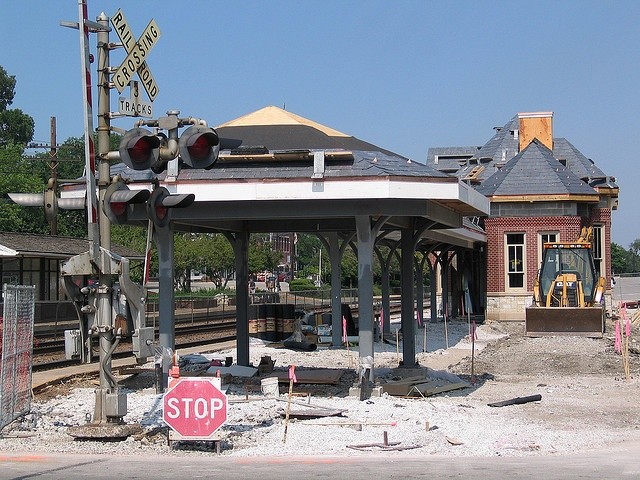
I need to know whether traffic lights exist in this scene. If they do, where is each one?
[100,181,150,227]
[147,185,196,228]
[177,124,221,168]
[118,127,161,171]
[53,190,99,221]
[7,189,58,221]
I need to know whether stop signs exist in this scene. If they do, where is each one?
[163,377,229,439]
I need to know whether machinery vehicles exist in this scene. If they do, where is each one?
[525,226,607,339]
